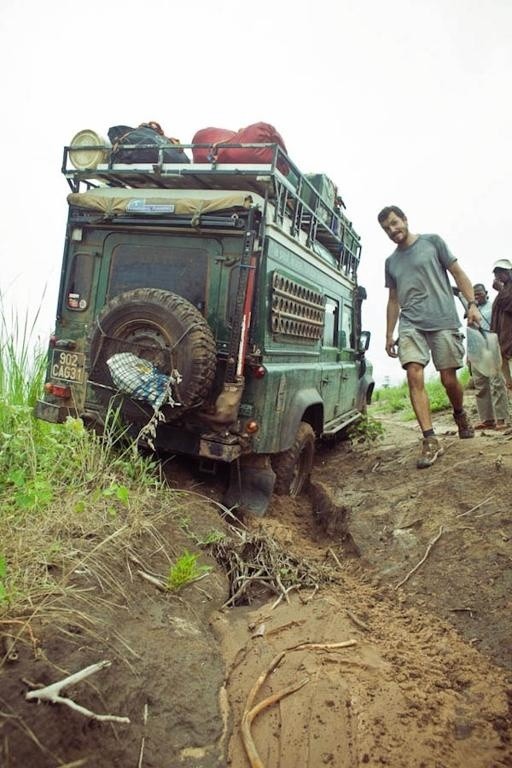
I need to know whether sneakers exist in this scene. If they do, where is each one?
[417,435,445,469]
[453,409,474,439]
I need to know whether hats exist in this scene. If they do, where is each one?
[491,259,512,272]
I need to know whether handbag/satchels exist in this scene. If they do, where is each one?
[190,123,289,191]
[266,167,360,270]
[107,126,190,188]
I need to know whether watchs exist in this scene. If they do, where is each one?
[467,301,479,307]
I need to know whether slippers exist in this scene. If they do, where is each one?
[472,422,508,430]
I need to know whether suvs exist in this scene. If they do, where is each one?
[34,142,375,521]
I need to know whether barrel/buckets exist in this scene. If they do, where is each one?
[69,130,113,170]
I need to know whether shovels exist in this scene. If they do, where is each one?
[451,286,503,378]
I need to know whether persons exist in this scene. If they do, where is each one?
[376,203,482,469]
[467,283,510,429]
[490,258,512,392]
